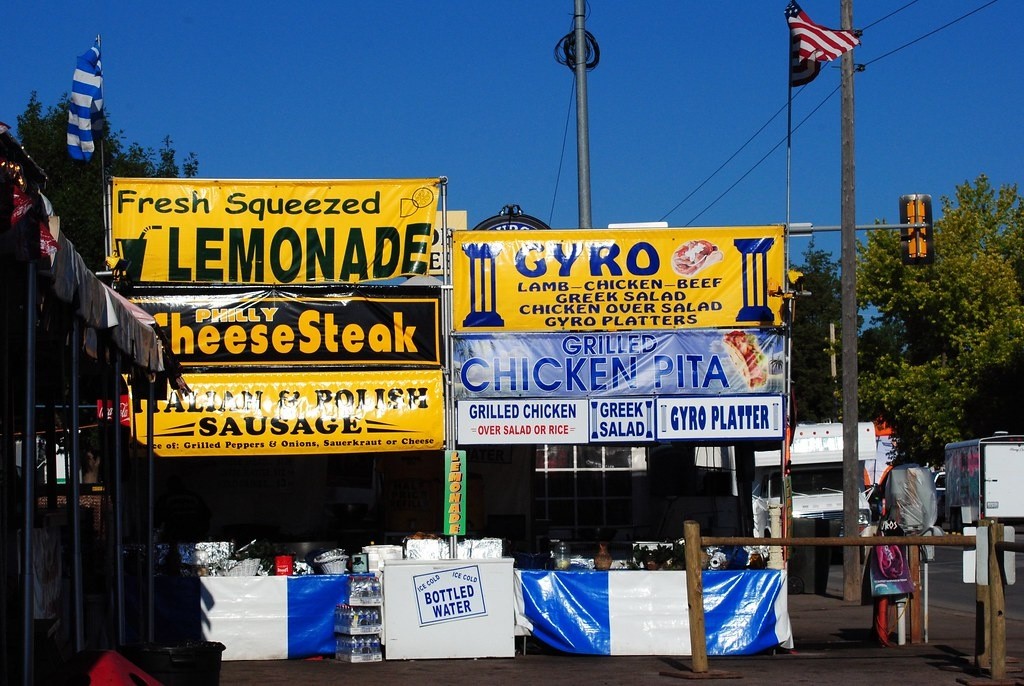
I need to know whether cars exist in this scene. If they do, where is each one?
[932,472,946,499]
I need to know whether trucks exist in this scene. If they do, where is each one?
[944,435,1024,535]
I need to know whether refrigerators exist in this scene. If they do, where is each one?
[383,558,515,658]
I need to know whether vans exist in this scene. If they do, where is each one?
[789,421,877,537]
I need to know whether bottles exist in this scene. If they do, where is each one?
[333,573,382,654]
[554,542,571,571]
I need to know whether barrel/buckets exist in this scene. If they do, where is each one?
[275,556,293,575]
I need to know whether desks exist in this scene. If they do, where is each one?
[515,568,794,655]
[201,572,381,660]
